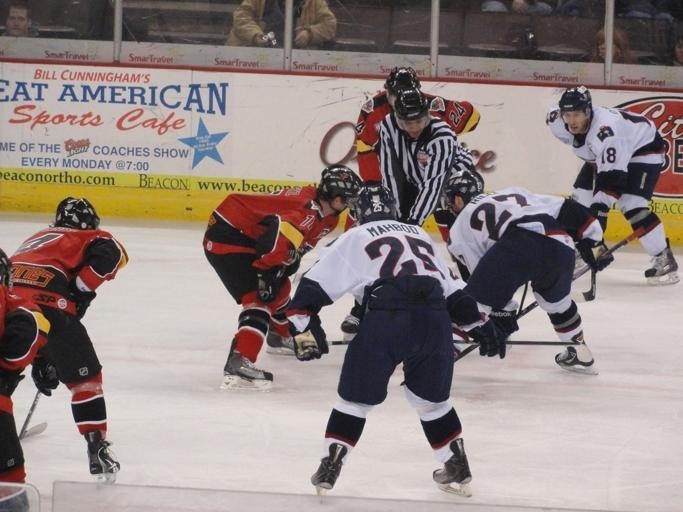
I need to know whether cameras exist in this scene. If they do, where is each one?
[263,31,279,46]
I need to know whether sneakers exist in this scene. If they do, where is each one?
[267,325,296,351]
[339,298,362,334]
[223,337,274,380]
[309,442,348,489]
[431,438,473,487]
[555,330,594,370]
[82,430,121,475]
[644,236,678,278]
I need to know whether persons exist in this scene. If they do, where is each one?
[225,0,336,51]
[1,251,52,511]
[467,1,682,67]
[202,164,362,381]
[345,68,480,243]
[2,1,38,37]
[547,86,679,275]
[443,170,614,367]
[282,182,506,489]
[9,197,130,474]
[341,87,473,333]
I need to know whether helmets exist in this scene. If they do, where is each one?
[385,63,430,121]
[442,168,486,214]
[55,196,100,229]
[559,86,594,127]
[320,164,398,222]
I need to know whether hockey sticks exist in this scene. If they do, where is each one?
[19,389,47,441]
[305,340,592,363]
[570,257,597,301]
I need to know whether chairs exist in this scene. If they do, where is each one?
[0,1,117,42]
[328,0,683,67]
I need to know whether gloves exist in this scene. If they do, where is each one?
[458,298,519,358]
[30,355,60,396]
[66,277,96,322]
[288,314,330,361]
[576,201,615,272]
